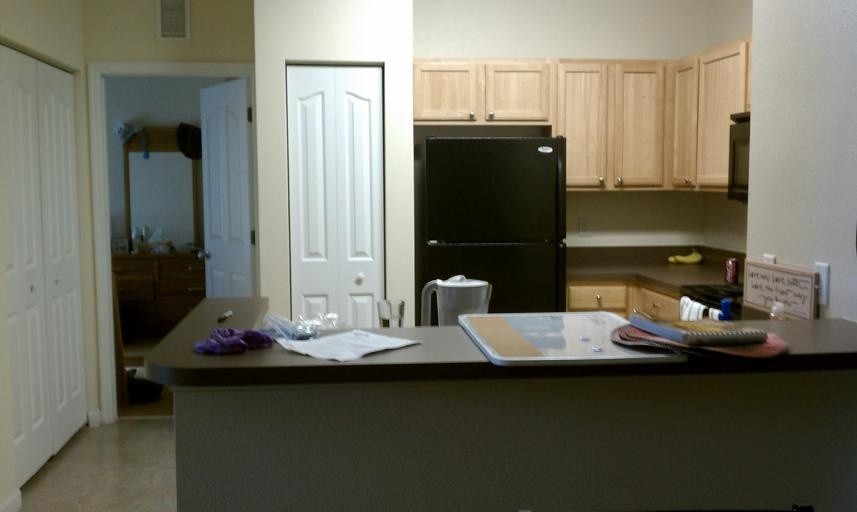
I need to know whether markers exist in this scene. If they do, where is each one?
[218,310,233,321]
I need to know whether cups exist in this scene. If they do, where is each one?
[767,301,787,321]
[376,298,405,331]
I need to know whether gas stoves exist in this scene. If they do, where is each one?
[679,280,744,311]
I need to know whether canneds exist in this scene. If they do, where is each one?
[725,258,739,283]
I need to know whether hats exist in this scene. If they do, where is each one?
[176,122,201,160]
[116,122,148,158]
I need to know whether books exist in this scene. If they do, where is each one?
[627,310,769,345]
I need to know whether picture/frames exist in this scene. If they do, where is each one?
[155,1,191,42]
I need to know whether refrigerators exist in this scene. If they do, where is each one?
[421,135,568,326]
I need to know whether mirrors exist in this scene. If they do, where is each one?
[123,127,202,255]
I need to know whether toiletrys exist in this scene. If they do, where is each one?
[141,222,153,242]
[131,226,141,251]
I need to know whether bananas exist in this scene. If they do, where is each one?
[667,248,703,264]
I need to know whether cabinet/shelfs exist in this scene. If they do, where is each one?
[413,59,553,127]
[109,255,203,331]
[566,281,679,321]
[555,39,749,192]
